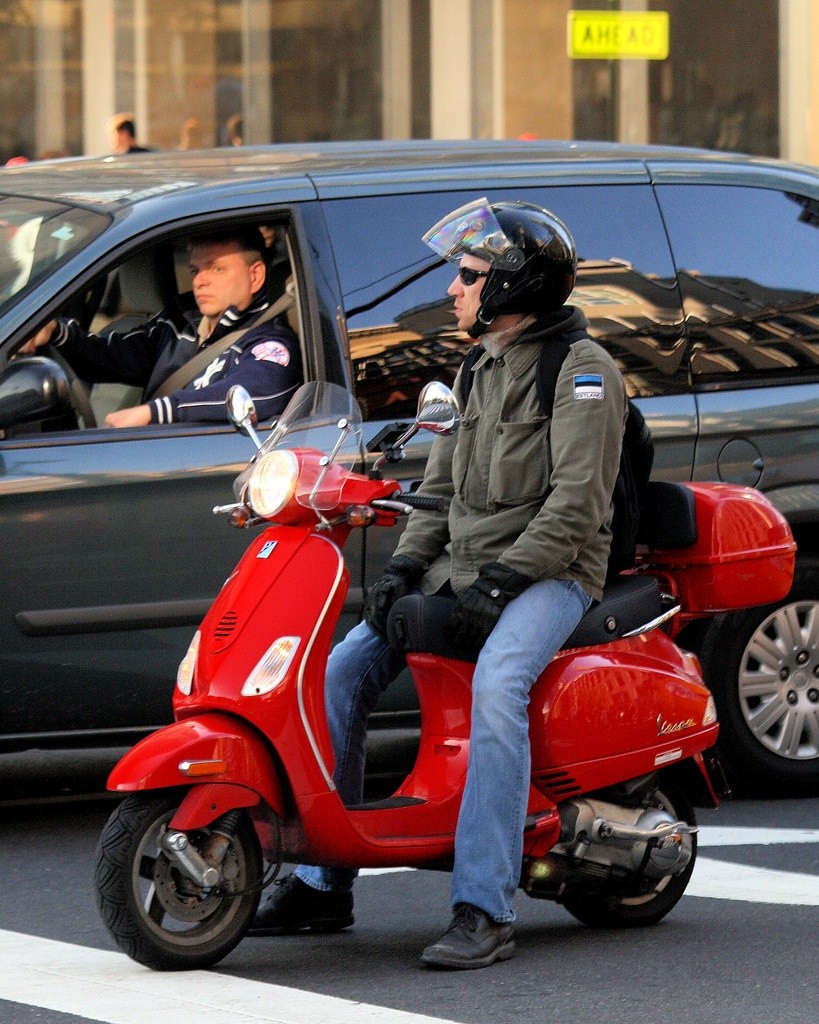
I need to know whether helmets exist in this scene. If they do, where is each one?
[421,197,578,318]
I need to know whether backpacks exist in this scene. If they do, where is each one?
[459,341,653,578]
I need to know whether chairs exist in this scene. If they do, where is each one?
[268,245,300,340]
[77,250,178,431]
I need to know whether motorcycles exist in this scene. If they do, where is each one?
[92,378,798,972]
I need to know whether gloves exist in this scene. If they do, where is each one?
[442,560,525,654]
[364,554,427,641]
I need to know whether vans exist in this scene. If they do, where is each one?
[1,137,819,810]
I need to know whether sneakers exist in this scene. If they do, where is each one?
[243,873,356,935]
[420,903,517,968]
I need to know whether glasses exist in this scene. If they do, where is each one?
[457,266,490,286]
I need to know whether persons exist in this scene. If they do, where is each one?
[243,198,627,969]
[9,224,299,429]
[178,114,242,151]
[0,143,75,295]
[111,115,150,152]
